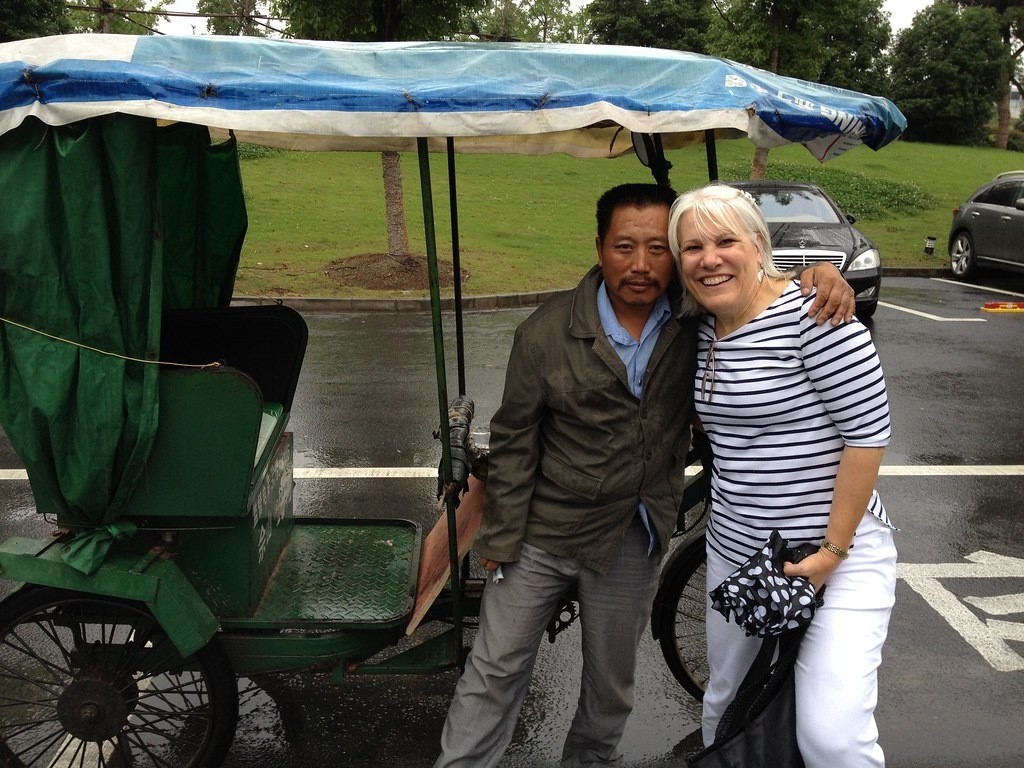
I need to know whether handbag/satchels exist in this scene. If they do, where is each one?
[686,627,806,768]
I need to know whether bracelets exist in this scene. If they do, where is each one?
[820,540,850,561]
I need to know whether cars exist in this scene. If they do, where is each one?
[947,170,1024,282]
[721,178,883,319]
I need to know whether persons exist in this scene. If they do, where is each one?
[432,184,855,768]
[668,184,901,768]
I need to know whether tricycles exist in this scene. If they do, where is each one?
[1,120,776,768]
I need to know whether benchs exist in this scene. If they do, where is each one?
[0,299,309,519]
